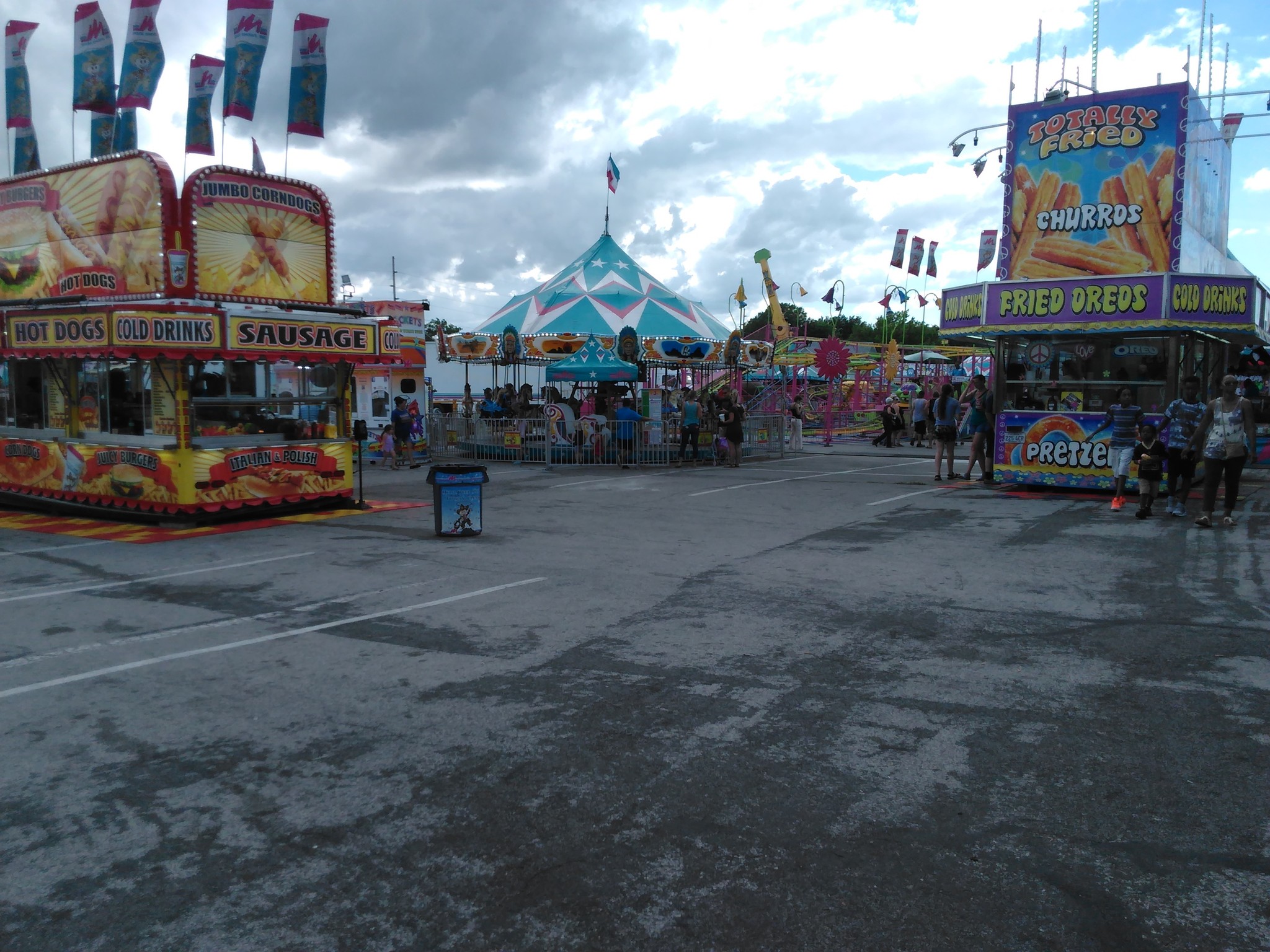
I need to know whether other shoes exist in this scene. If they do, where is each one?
[524,455,530,461]
[514,461,521,464]
[618,455,622,467]
[675,465,682,467]
[799,447,803,450]
[622,465,630,469]
[789,448,794,450]
[580,461,582,464]
[984,479,1001,485]
[872,438,936,449]
[390,465,400,468]
[735,464,740,467]
[693,464,696,468]
[379,466,388,470]
[409,465,421,469]
[391,466,400,470]
[724,464,735,468]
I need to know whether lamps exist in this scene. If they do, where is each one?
[1042,79,1099,107]
[340,275,356,303]
[949,123,1008,157]
[971,146,1007,178]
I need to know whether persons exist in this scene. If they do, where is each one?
[714,385,746,468]
[1181,374,1257,527]
[391,394,421,469]
[573,420,584,464]
[380,425,399,471]
[958,374,998,484]
[788,395,804,450]
[615,398,653,469]
[1154,376,1208,517]
[592,425,603,466]
[1084,384,1146,512]
[872,395,905,448]
[675,390,702,467]
[513,409,528,464]
[952,361,967,401]
[909,390,940,449]
[932,384,961,481]
[1132,424,1168,522]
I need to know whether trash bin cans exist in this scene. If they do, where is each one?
[426,463,489,537]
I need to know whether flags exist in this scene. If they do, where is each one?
[5,0,330,176]
[908,236,925,278]
[977,229,1003,278]
[606,156,621,195]
[927,241,939,278]
[890,229,910,269]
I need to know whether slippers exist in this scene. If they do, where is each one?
[1195,516,1212,526]
[1220,516,1237,526]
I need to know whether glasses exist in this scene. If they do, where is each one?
[894,399,899,401]
[1223,380,1239,387]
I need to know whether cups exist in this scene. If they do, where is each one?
[167,250,190,287]
[62,444,84,492]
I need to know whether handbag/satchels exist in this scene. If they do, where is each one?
[1225,440,1245,458]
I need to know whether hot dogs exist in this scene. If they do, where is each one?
[53,441,88,477]
[243,465,306,497]
[45,208,103,276]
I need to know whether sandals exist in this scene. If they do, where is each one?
[975,476,985,481]
[947,471,961,480]
[958,473,971,480]
[934,475,942,480]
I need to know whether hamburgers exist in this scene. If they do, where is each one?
[110,464,144,499]
[0,209,42,299]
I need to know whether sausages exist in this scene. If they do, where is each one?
[241,214,289,278]
[94,162,154,276]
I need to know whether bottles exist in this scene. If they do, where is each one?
[298,419,338,440]
[894,413,898,419]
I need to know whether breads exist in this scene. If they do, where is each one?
[0,454,56,485]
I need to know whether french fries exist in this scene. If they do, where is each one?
[31,256,59,298]
[143,485,178,503]
[195,482,254,502]
[296,475,345,493]
[127,249,164,292]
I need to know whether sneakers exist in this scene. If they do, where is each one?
[1111,496,1186,519]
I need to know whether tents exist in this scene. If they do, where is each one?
[546,334,638,469]
